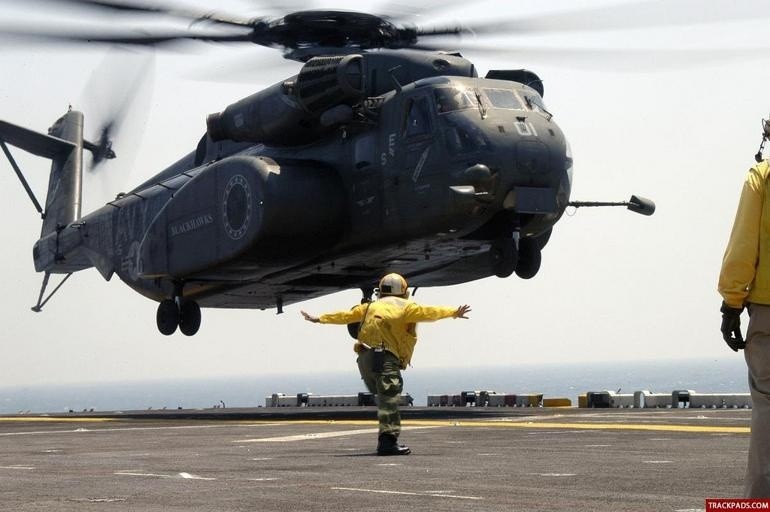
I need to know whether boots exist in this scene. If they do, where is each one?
[377,434,411,456]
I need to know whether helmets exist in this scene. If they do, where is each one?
[379,272,409,300]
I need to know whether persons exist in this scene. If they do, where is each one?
[299,272,471,458]
[718,119,770,503]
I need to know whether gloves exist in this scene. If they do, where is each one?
[721,302,745,351]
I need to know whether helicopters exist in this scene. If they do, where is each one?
[0,0,770,339]
